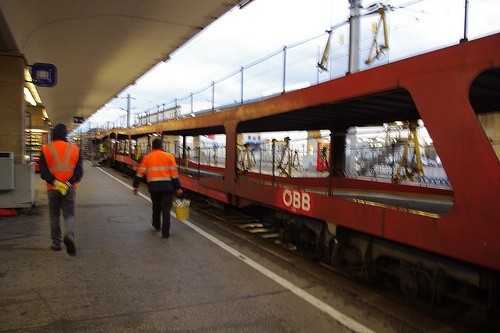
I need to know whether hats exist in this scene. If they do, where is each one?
[54,124,68,143]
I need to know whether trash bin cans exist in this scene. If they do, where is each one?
[175,204,191,221]
[0,151,35,209]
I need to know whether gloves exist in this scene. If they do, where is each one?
[52,180,69,196]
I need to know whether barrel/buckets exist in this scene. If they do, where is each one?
[173,196,191,221]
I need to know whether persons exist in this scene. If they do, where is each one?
[132,139,183,239]
[39,124,84,258]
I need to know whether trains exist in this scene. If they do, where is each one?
[89,28,500,301]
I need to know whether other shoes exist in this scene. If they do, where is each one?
[152,223,161,231]
[162,232,170,239]
[63,237,77,256]
[50,246,61,251]
